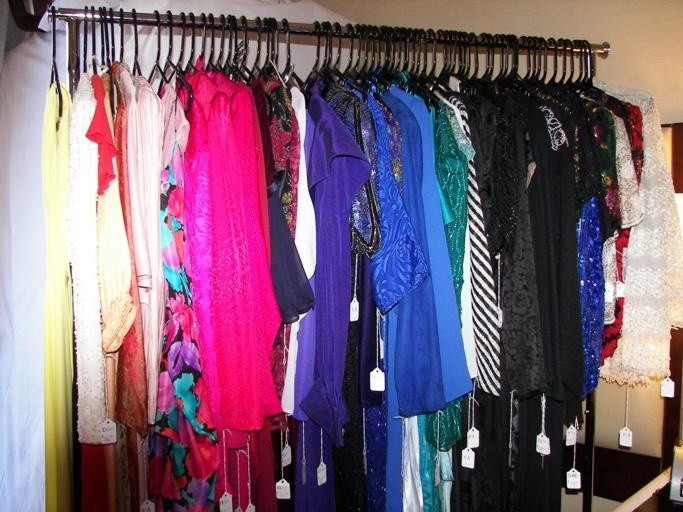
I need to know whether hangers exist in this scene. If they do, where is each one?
[49,5,61,121]
